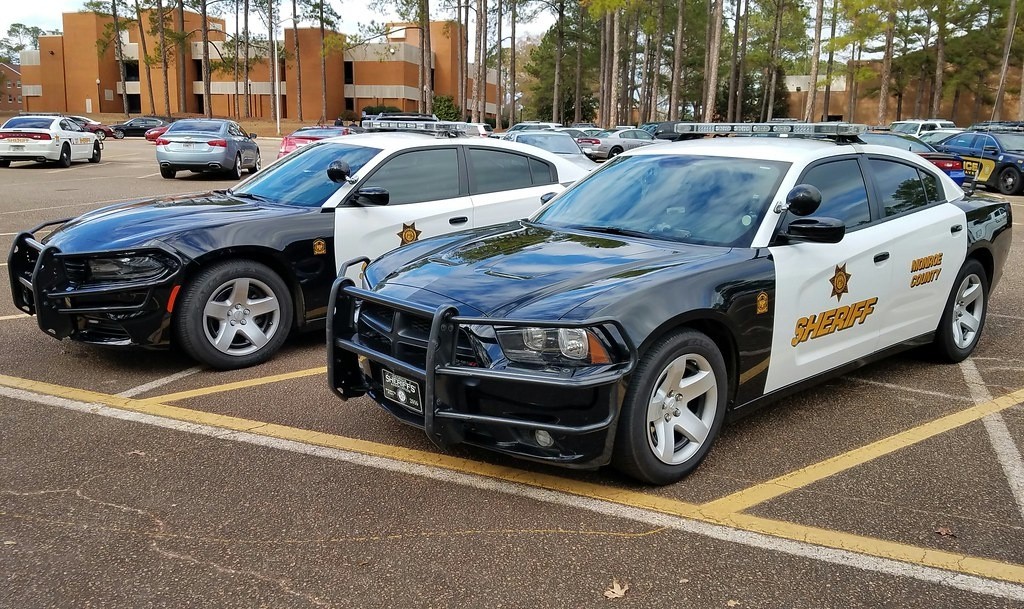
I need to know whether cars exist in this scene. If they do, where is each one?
[279,112,1024,198]
[106,117,171,140]
[324,120,1015,486]
[155,118,262,181]
[144,121,175,143]
[0,115,104,168]
[68,115,115,141]
[6,120,587,371]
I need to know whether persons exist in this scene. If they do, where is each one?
[349,120,358,127]
[335,116,343,126]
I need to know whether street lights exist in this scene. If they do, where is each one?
[275,17,300,136]
[96,78,102,113]
